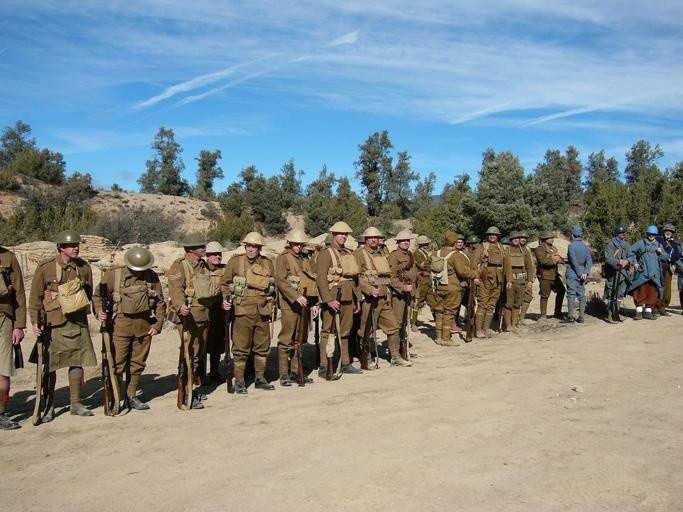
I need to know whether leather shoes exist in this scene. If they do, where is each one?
[42,405,54,422]
[341,364,361,372]
[560,318,574,323]
[71,403,93,417]
[436,339,460,346]
[391,358,412,367]
[255,377,274,389]
[319,367,328,378]
[644,312,657,320]
[234,382,246,392]
[124,397,150,410]
[0,414,19,430]
[184,389,207,409]
[634,311,643,320]
[279,372,312,386]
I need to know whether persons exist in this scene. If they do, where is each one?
[564,223,683,322]
[92,248,166,411]
[0,245,27,430]
[168,221,568,409]
[28,230,97,423]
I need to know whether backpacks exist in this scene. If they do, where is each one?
[432,250,455,285]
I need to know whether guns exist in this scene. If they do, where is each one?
[465,279,473,342]
[2,270,24,369]
[31,306,50,425]
[224,294,236,393]
[400,280,412,361]
[361,285,378,369]
[295,288,308,387]
[178,279,186,410]
[100,282,113,415]
[326,289,341,379]
[497,261,507,332]
[610,263,621,321]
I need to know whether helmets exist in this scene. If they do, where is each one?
[486,226,529,239]
[244,232,265,245]
[467,235,478,244]
[394,231,414,239]
[663,224,674,231]
[541,231,553,238]
[182,232,226,253]
[124,247,154,271]
[285,229,308,243]
[330,221,353,233]
[416,236,432,245]
[55,230,81,244]
[646,225,658,234]
[362,227,381,237]
[457,233,464,240]
[615,226,625,234]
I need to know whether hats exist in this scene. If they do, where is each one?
[572,226,582,237]
[444,230,457,245]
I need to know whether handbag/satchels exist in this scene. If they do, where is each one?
[119,285,150,315]
[299,270,319,297]
[247,264,269,291]
[58,278,91,314]
[338,255,359,276]
[488,252,502,266]
[373,257,391,275]
[509,253,524,268]
[192,274,217,300]
